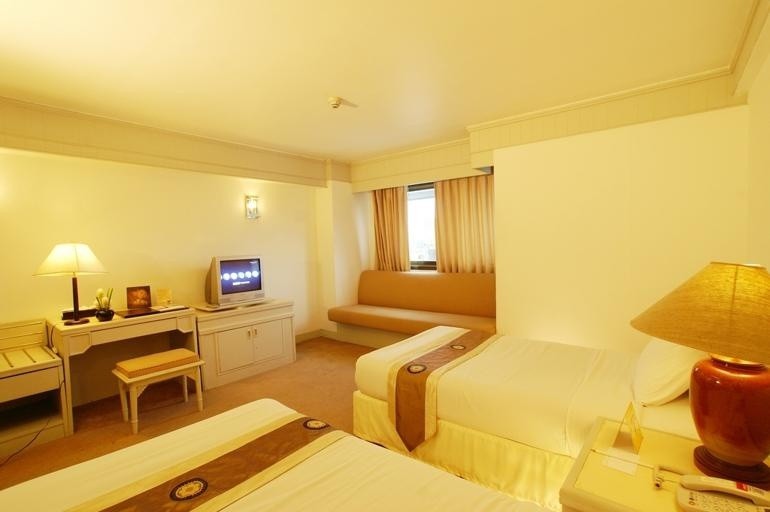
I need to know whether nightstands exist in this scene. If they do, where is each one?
[560,417,770,512]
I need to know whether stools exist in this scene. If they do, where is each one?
[112,348,205,423]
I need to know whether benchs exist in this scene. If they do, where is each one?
[328,269,496,348]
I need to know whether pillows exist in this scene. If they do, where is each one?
[633,337,711,407]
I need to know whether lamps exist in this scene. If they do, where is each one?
[244,194,261,221]
[31,243,108,326]
[629,260,770,490]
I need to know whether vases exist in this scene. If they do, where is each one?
[95,310,114,321]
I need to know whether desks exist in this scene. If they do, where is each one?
[48,303,199,434]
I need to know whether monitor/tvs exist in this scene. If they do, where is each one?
[205,255,265,305]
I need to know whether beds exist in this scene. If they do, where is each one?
[0,398,550,512]
[354,324,702,512]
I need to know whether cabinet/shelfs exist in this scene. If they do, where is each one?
[0,320,69,462]
[197,302,297,390]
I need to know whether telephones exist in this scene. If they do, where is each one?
[675,475,770,512]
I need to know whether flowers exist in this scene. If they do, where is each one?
[93,288,113,310]
